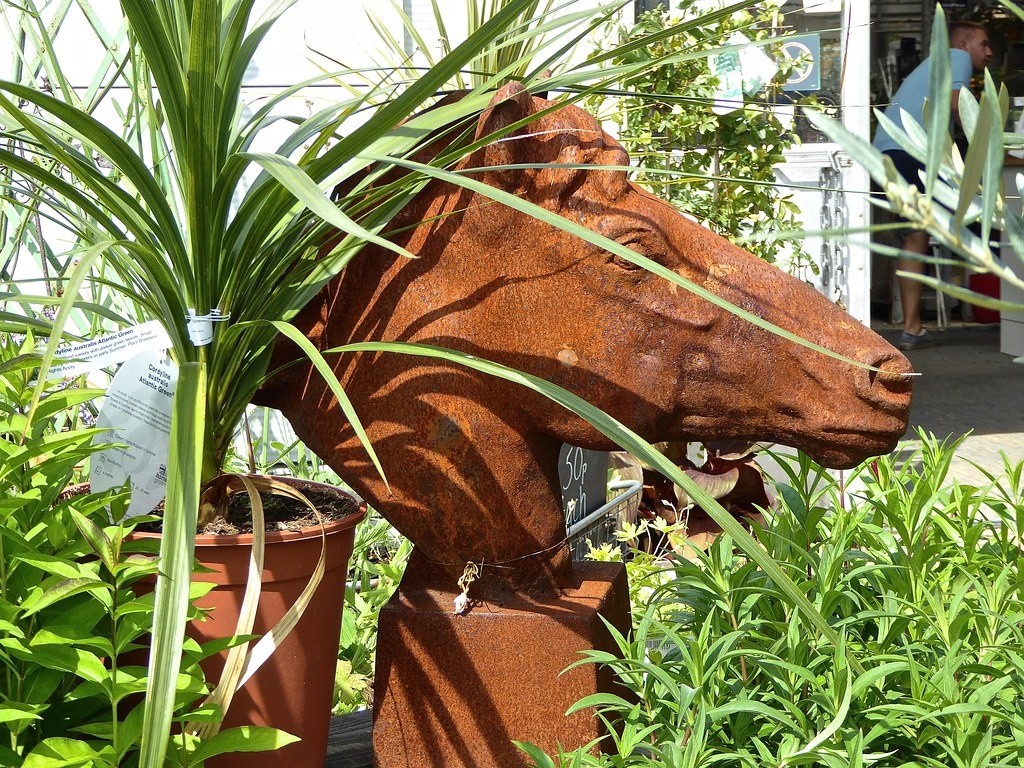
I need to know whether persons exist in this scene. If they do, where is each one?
[873,23,993,351]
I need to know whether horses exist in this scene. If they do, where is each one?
[247,66,916,599]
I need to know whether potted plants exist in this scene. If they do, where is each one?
[0,0,858,768]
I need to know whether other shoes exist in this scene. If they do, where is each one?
[900,327,943,350]
